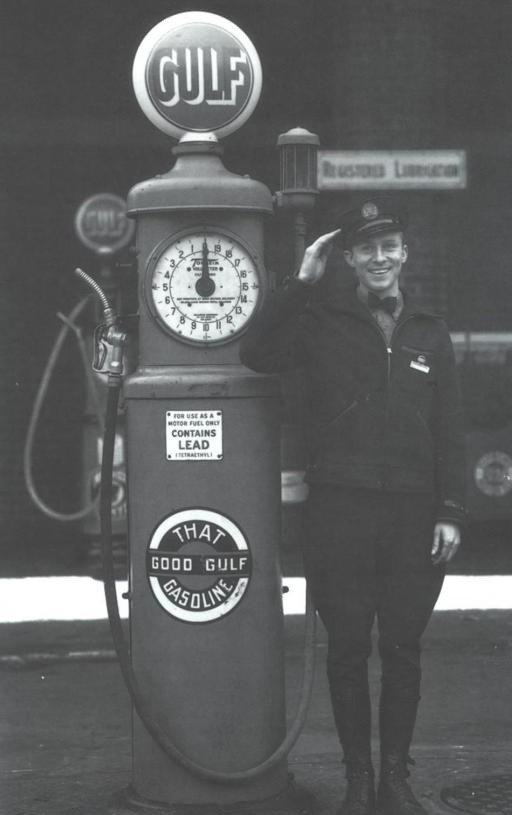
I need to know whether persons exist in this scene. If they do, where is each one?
[237,187,476,815]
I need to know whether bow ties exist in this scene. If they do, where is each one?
[366,290,399,313]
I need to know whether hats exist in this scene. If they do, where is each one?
[333,193,413,248]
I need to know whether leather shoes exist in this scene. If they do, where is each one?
[378,758,430,815]
[333,764,378,815]
